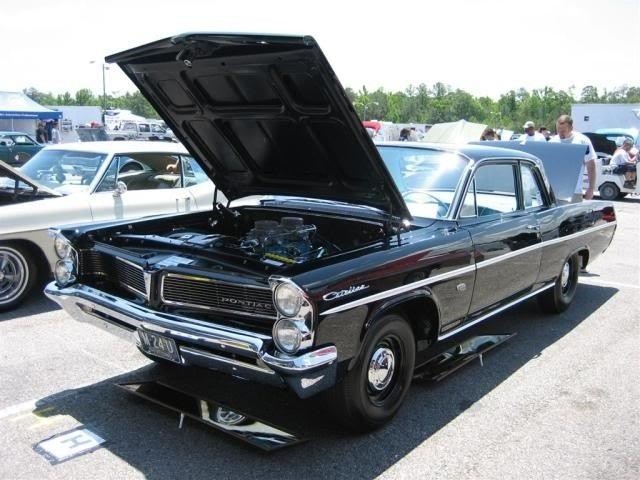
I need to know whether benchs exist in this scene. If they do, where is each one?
[125,173,194,189]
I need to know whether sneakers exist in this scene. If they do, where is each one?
[623,180,636,189]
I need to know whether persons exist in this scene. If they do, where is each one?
[609,137,639,188]
[51,121,62,144]
[34,120,49,143]
[546,114,598,200]
[479,120,551,142]
[399,124,432,143]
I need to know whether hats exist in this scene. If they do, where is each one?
[522,121,535,128]
